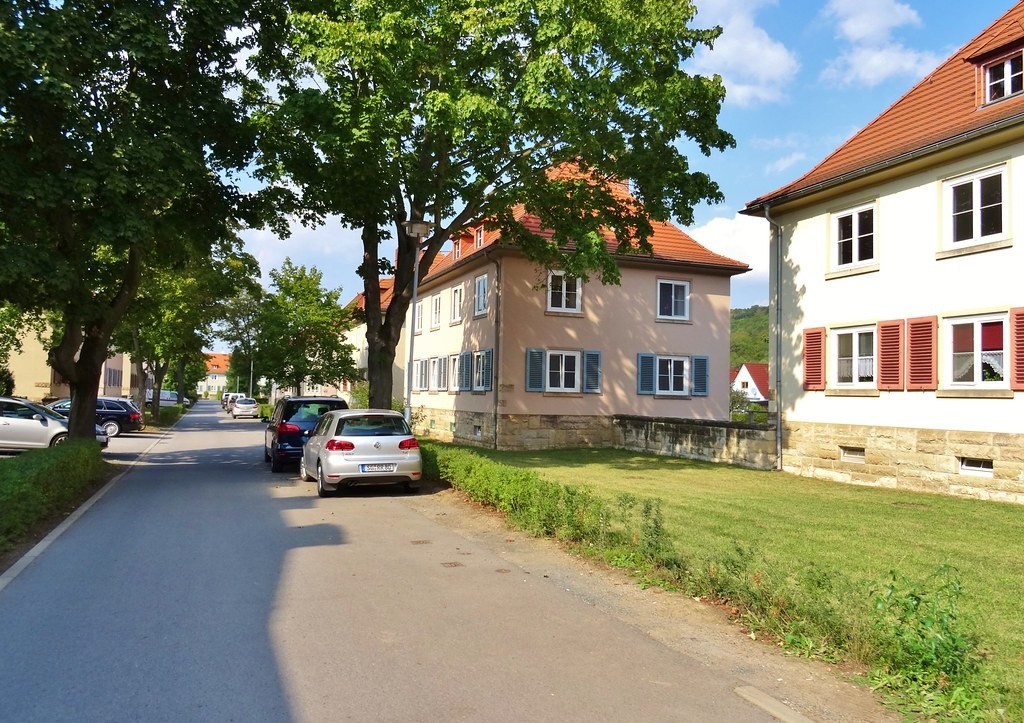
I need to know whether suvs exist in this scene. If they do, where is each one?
[260,395,349,475]
[47,397,144,438]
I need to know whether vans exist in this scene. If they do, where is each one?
[146,389,191,408]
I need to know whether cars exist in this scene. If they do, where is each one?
[232,398,259,419]
[299,408,424,498]
[221,392,245,414]
[0,397,111,453]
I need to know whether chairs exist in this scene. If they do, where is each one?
[318,407,329,420]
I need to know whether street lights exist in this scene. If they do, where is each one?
[400,219,436,431]
